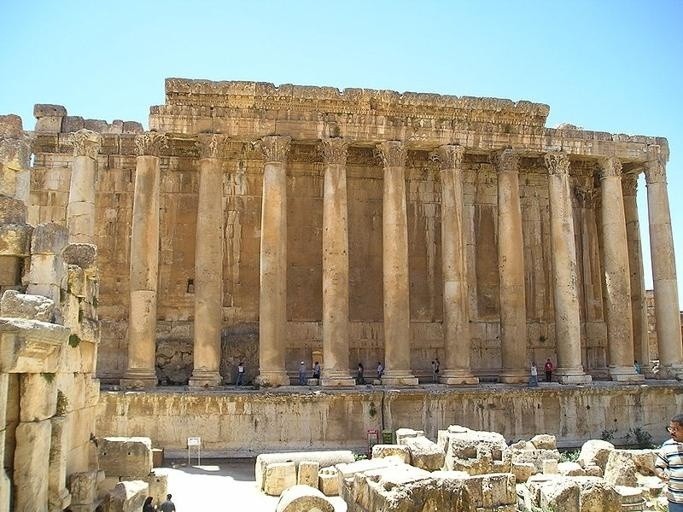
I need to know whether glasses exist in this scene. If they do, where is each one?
[666,425,682,433]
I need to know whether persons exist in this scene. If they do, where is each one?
[530,361,540,387]
[545,358,553,382]
[313,361,320,379]
[635,360,641,373]
[143,497,157,512]
[159,494,176,512]
[654,414,683,512]
[376,362,383,379]
[298,361,306,385]
[432,358,441,382]
[358,363,364,385]
[236,363,245,386]
